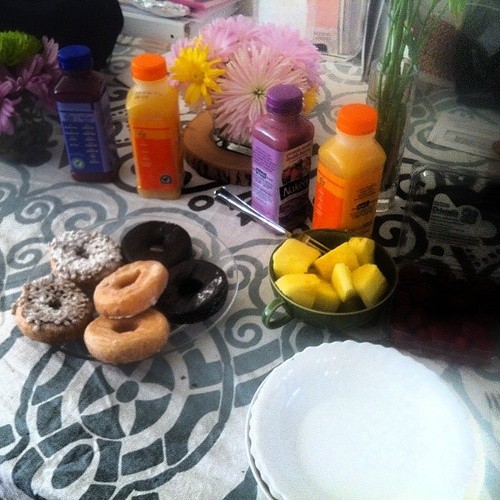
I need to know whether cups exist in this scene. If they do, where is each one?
[262,228,398,329]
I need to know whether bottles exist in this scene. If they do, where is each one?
[127,52,183,199]
[52,45,120,182]
[310,103,387,240]
[249,84,314,235]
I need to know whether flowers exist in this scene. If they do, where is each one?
[165,14,325,145]
[0,31,64,137]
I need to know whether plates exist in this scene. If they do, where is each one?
[27,214,239,359]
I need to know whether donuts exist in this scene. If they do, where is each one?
[11,220,228,364]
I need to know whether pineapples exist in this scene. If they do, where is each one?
[272,237,388,313]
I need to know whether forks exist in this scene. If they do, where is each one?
[215,187,332,255]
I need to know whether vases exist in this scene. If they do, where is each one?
[367,58,417,212]
[183,109,252,187]
